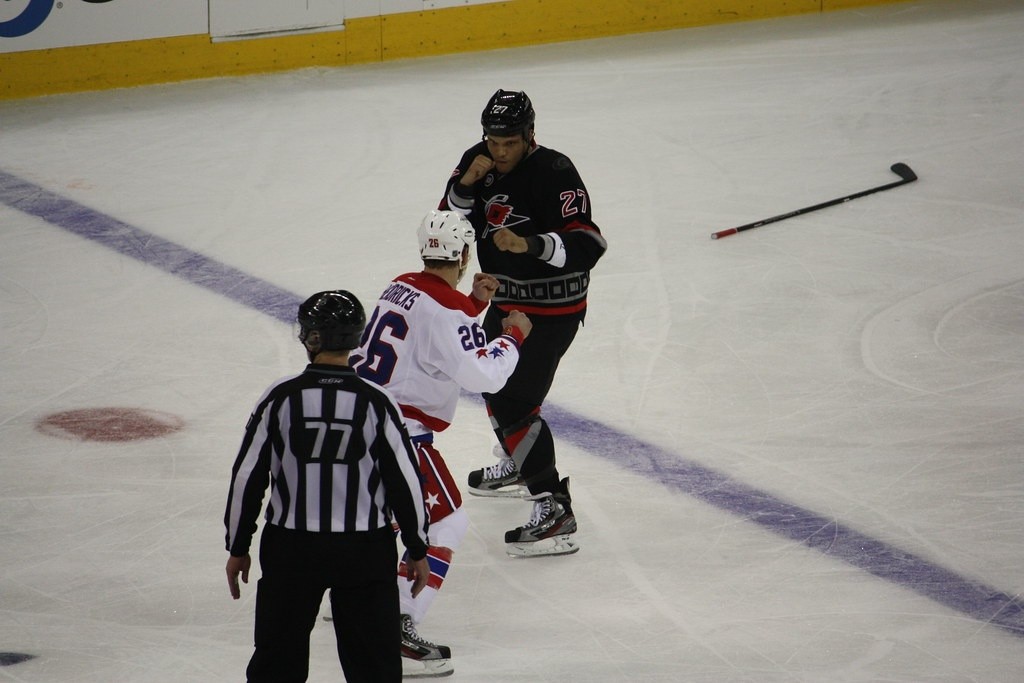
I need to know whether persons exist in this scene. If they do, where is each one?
[437,89,607,558]
[323,210,532,679]
[223,289,429,683]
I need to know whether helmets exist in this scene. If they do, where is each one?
[297,290,366,350]
[480,89,535,137]
[416,209,476,261]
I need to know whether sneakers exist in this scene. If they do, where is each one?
[504,476,580,559]
[467,441,532,498]
[399,614,454,678]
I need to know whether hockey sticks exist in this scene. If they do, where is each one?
[709,161,919,240]
